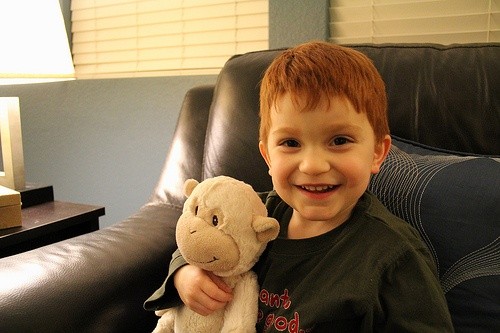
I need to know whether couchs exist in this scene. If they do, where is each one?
[0,42,499,332]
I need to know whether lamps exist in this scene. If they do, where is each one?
[1,0,77,84]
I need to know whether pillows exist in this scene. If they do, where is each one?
[369,132,500,333]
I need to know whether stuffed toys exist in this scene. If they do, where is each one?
[153,174,280,333]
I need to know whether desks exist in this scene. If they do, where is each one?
[0,200,106,260]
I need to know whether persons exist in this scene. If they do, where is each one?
[142,40,457,332]
